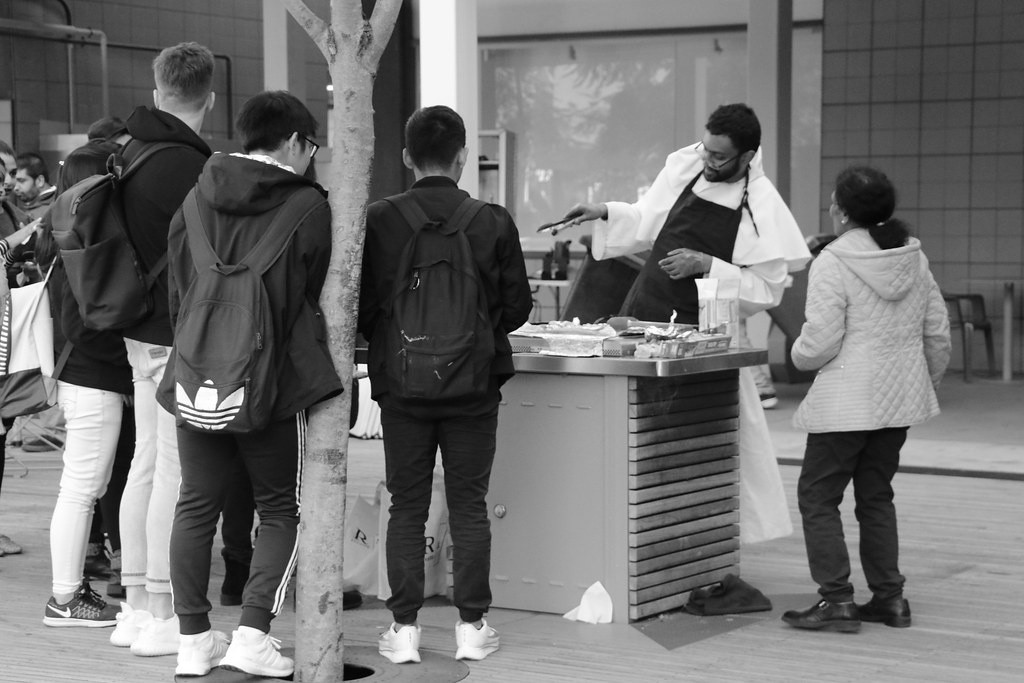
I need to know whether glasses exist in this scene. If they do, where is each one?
[696,142,738,171]
[298,131,320,159]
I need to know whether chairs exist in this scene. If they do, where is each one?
[939,289,997,385]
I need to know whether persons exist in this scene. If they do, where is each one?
[359,103,531,664]
[560,103,792,547]
[155,92,341,676]
[103,42,217,656]
[781,167,953,634]
[0,108,143,639]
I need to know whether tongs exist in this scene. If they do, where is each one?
[536,215,585,235]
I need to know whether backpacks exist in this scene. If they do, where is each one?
[52,139,194,333]
[172,187,328,432]
[381,191,494,403]
[0,256,72,419]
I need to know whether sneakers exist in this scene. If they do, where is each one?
[218,629,296,678]
[109,601,181,657]
[43,582,122,627]
[378,622,422,663]
[174,631,229,677]
[454,615,501,661]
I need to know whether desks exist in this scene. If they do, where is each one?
[528,279,572,323]
[436,343,770,625]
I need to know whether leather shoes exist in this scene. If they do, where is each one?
[858,598,912,628]
[83,552,127,598]
[781,599,862,633]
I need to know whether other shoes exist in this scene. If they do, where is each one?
[0,534,23,556]
[219,558,249,605]
[22,436,63,452]
